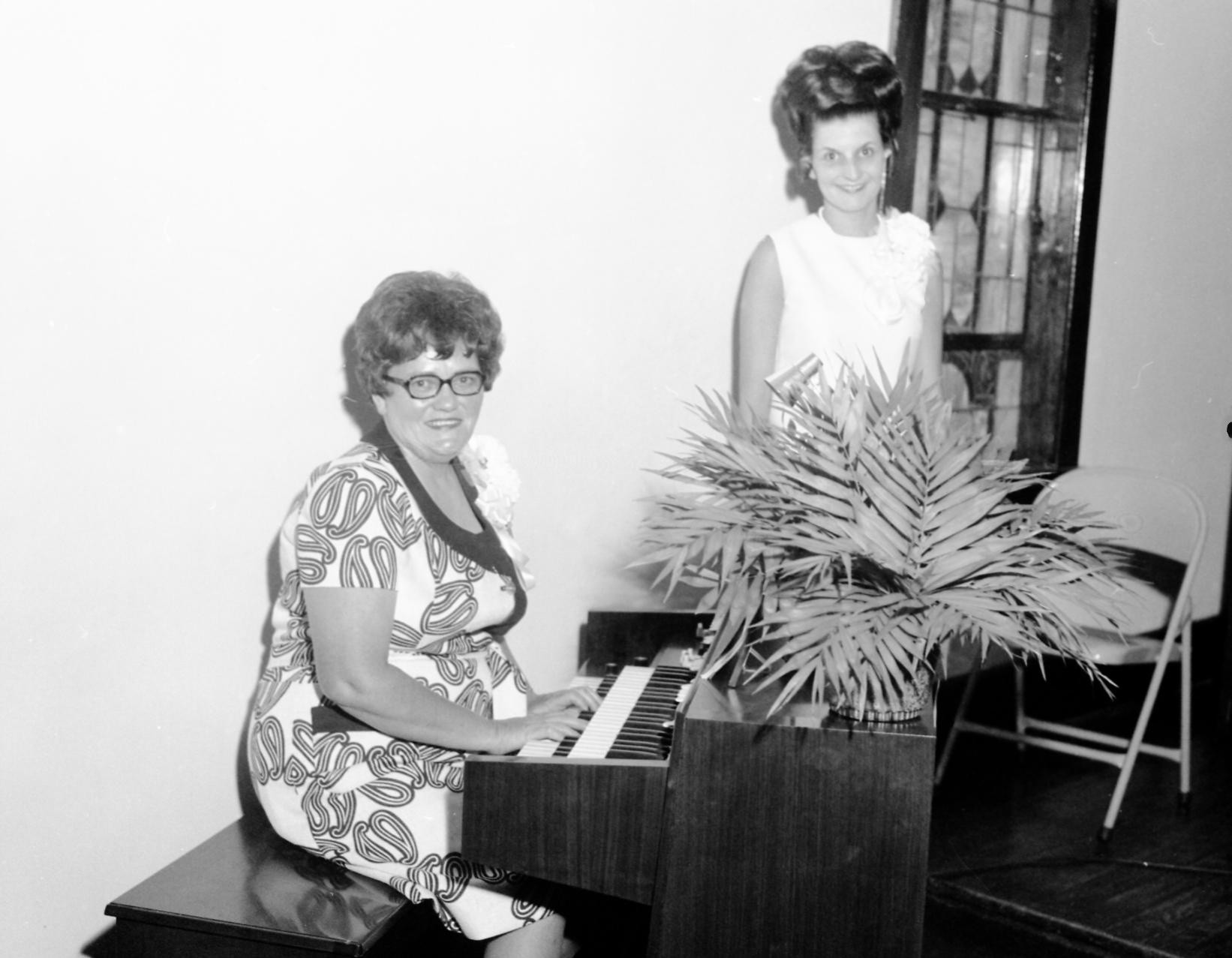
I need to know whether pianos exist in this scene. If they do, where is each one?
[460,606,939,958]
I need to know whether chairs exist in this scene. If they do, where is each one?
[934,466,1211,840]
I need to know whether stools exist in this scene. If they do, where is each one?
[104,804,477,958]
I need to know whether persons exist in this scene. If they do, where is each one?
[736,42,945,442]
[247,270,604,957]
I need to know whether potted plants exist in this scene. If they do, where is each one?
[626,332,1159,724]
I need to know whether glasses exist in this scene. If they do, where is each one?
[380,370,487,400]
